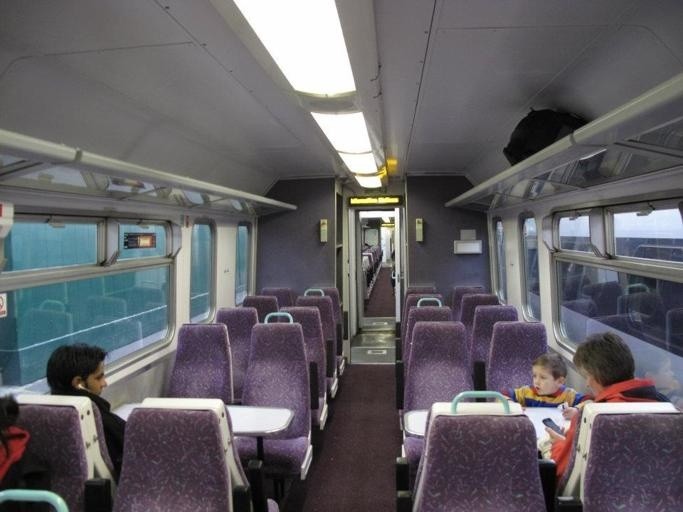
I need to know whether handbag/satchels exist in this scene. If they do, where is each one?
[501,108,585,167]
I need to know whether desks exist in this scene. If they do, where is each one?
[114,400,294,465]
[401,403,572,479]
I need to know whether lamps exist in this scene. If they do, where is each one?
[232,0,384,190]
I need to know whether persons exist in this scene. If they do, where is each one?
[544,332,670,487]
[0,396,53,512]
[495,352,594,419]
[46,341,128,478]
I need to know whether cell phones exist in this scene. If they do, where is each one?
[543,418,565,436]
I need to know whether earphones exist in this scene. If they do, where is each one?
[78,384,89,391]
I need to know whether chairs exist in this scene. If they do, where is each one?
[400,282,550,455]
[120,397,249,510]
[361,245,382,288]
[408,402,551,510]
[6,391,114,512]
[530,272,683,356]
[555,399,683,510]
[167,284,349,480]
[9,280,170,385]
